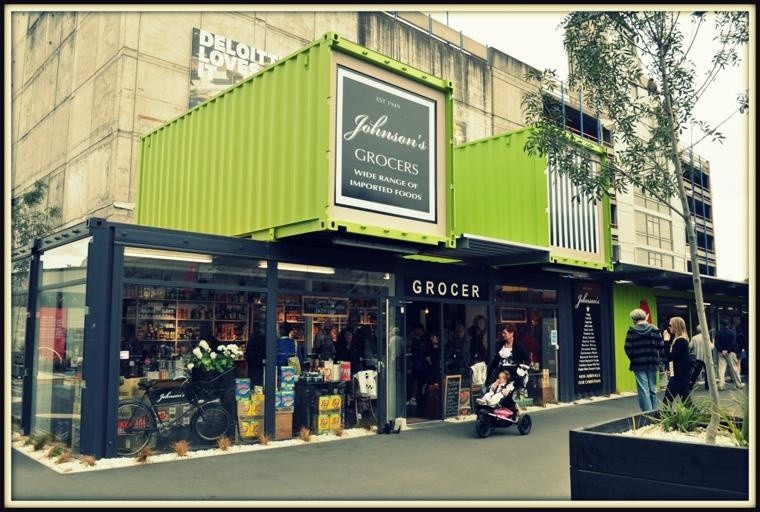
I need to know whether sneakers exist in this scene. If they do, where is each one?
[405,399,418,406]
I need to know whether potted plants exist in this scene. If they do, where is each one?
[525,10,747,502]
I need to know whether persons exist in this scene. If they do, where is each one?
[662,316,700,412]
[476,370,515,408]
[485,323,533,389]
[714,319,746,392]
[244,313,488,419]
[623,308,671,412]
[689,324,715,391]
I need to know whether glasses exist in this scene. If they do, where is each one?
[668,324,673,328]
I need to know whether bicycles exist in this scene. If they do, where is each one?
[116,367,235,457]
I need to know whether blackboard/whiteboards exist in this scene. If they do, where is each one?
[444,375,461,414]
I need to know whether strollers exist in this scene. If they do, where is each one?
[473,363,532,438]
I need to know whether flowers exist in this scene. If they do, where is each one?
[186,340,240,376]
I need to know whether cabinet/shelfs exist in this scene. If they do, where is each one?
[120,297,249,381]
[252,296,377,347]
